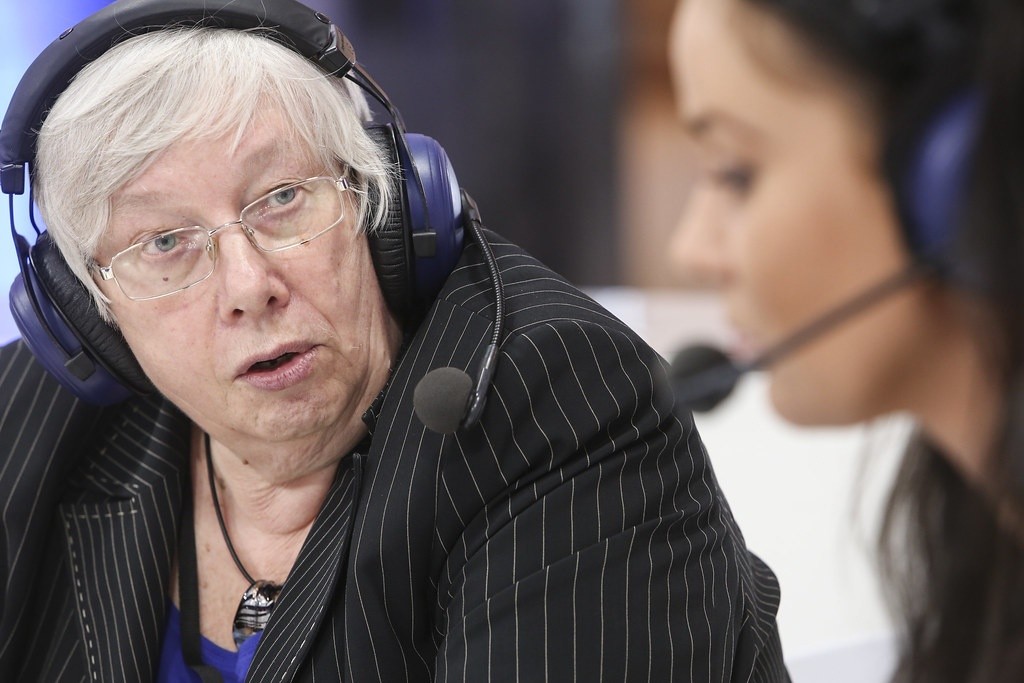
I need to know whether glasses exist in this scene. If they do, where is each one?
[87,163,350,301]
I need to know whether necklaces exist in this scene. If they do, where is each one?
[204,432,286,651]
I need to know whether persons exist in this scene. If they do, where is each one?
[0,0,794,683]
[667,0,1024,683]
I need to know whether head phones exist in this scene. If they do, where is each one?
[0,0,484,407]
[854,0,1023,285]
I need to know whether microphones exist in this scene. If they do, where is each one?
[413,220,505,434]
[669,260,935,412]
